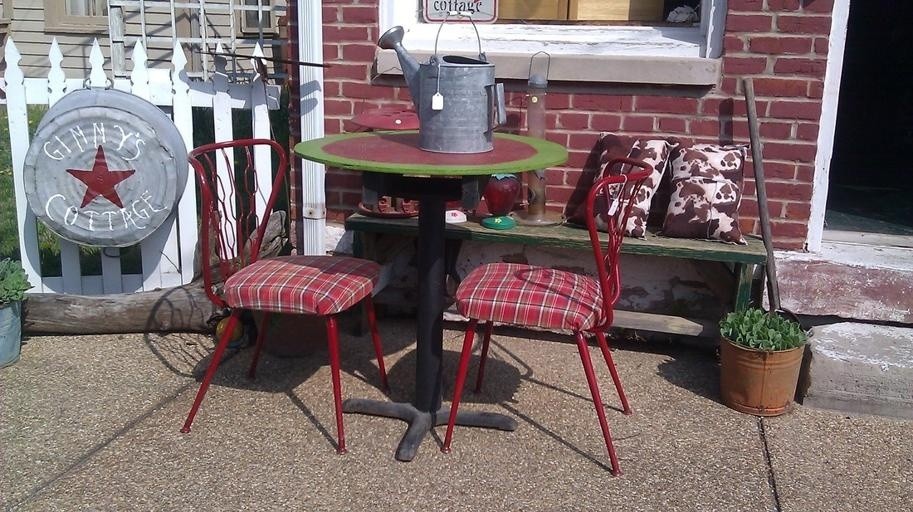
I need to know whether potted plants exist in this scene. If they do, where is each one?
[716,302,807,418]
[0,257,33,372]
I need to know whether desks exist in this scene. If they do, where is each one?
[296,127,586,461]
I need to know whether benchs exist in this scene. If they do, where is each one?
[341,201,768,359]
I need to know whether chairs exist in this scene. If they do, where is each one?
[434,157,652,478]
[178,140,395,455]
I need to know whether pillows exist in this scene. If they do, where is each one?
[664,136,749,245]
[561,132,678,241]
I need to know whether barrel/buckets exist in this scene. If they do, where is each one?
[0,300,22,368]
[719,327,806,418]
